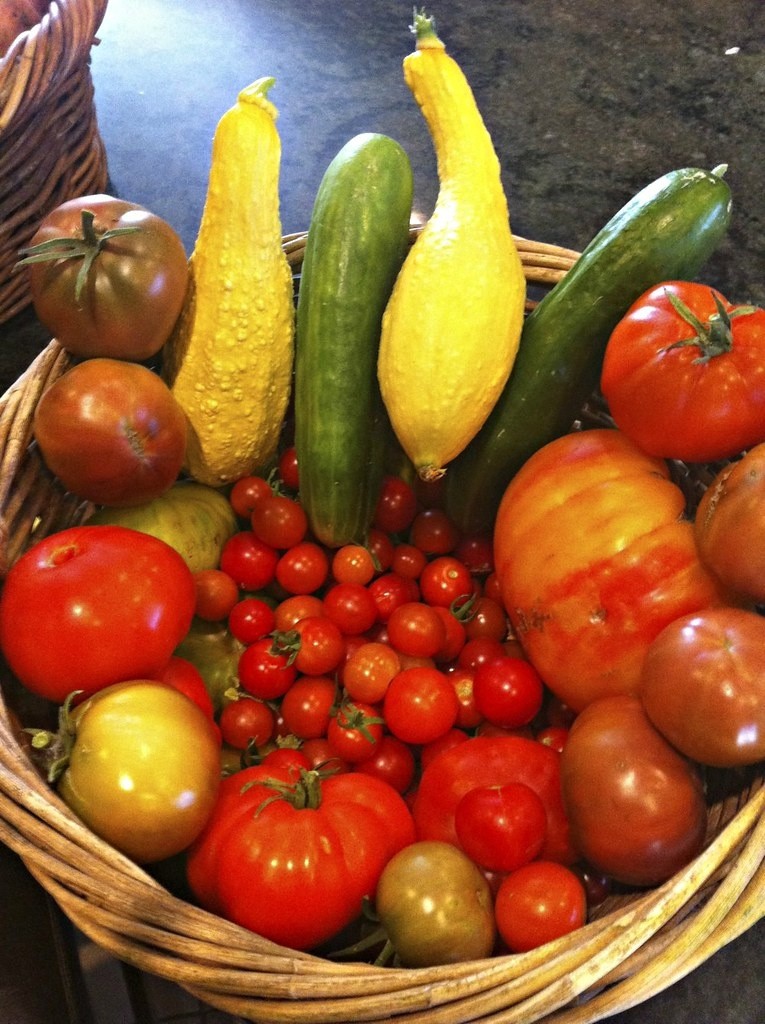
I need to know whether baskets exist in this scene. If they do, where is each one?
[0,224,765,1024]
[0,0,109,329]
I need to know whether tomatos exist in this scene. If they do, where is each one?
[0,194,765,967]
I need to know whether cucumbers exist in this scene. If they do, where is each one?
[292,131,414,544]
[445,162,734,541]
